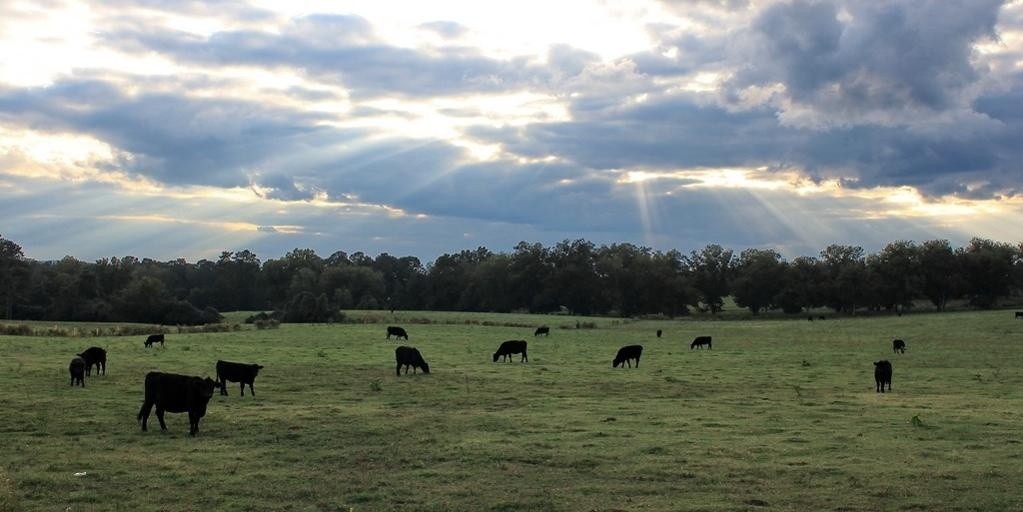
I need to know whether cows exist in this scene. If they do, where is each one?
[612,345,643,368]
[216,360,264,396]
[493,340,528,363]
[690,336,712,350]
[137,372,221,438]
[387,326,408,340]
[144,334,164,348]
[873,360,892,393]
[69,358,85,388]
[535,327,549,336]
[657,330,662,337]
[893,339,905,353]
[395,346,430,376]
[1015,312,1023,318]
[76,346,107,377]
